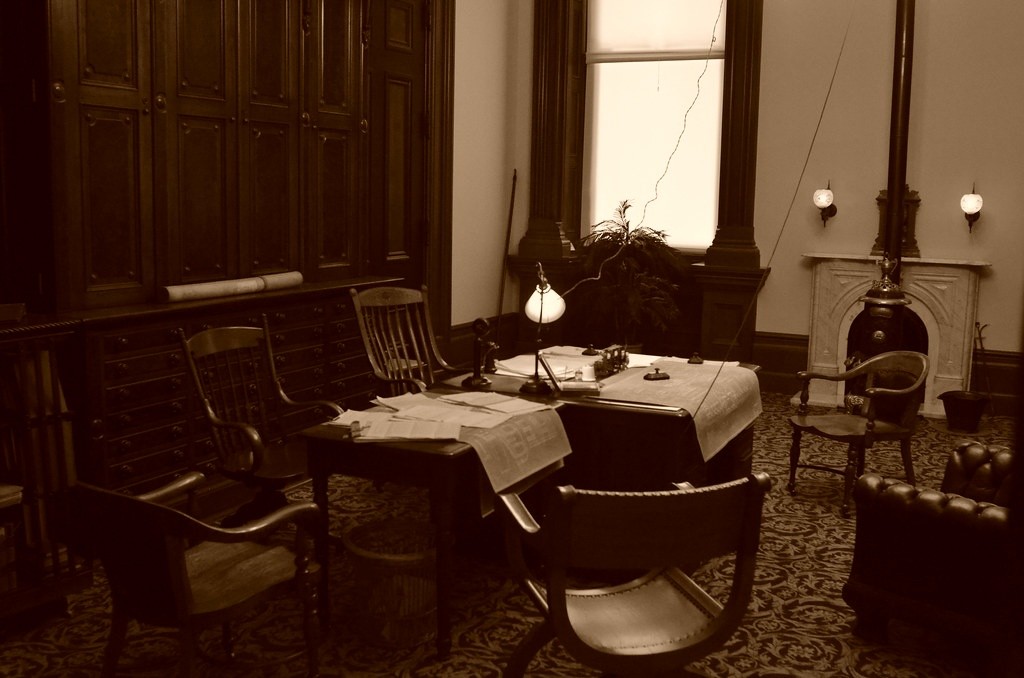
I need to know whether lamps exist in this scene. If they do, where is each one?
[961,181,984,234]
[812,179,837,229]
[519,261,567,396]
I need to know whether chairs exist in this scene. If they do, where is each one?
[786,350,930,512]
[293,514,453,678]
[349,282,475,392]
[504,472,773,678]
[69,470,323,678]
[175,312,345,487]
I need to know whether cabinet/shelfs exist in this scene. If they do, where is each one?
[0,0,455,551]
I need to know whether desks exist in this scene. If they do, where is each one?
[303,383,566,587]
[438,343,762,570]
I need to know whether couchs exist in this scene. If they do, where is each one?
[842,439,1015,665]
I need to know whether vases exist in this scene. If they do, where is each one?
[936,390,991,432]
